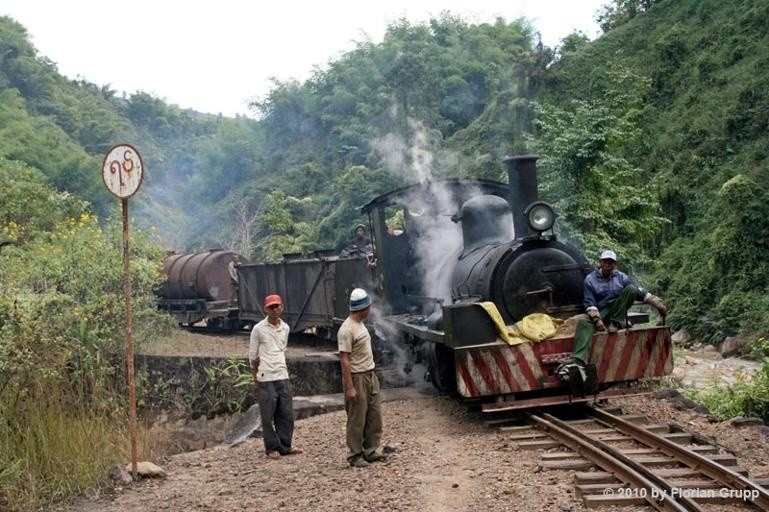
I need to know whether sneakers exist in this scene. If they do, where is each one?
[556,357,583,365]
[608,321,619,334]
[267,446,388,467]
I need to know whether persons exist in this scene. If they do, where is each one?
[563,250,668,365]
[336,287,386,469]
[341,223,375,258]
[247,295,303,459]
[385,220,405,235]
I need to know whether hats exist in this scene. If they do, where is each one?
[265,295,282,307]
[600,250,617,262]
[350,288,372,311]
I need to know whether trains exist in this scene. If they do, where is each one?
[150,154,675,416]
